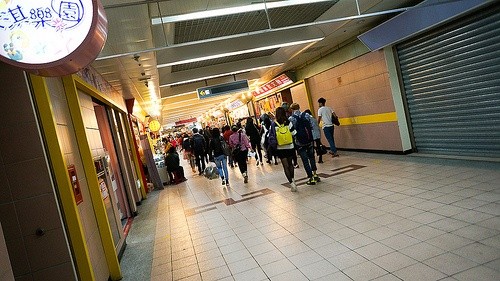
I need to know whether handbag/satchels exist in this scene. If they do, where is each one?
[218,136,231,156]
[316,143,328,156]
[230,145,240,160]
[203,162,220,180]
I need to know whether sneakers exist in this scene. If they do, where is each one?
[313,174,320,182]
[306,179,315,185]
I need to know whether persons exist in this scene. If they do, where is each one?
[245,114,279,166]
[162,136,184,182]
[305,109,324,163]
[182,118,242,175]
[270,107,298,192]
[208,128,229,186]
[282,103,301,169]
[288,103,321,185]
[317,98,339,158]
[229,125,254,183]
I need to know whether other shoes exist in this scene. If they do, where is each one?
[266,161,271,164]
[289,178,298,192]
[260,162,263,165]
[222,180,225,185]
[293,164,299,168]
[227,161,238,168]
[329,151,339,158]
[274,162,278,165]
[318,161,323,164]
[198,170,204,176]
[256,160,259,166]
[170,177,188,185]
[226,180,230,186]
[242,174,249,183]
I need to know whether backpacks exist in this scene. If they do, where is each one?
[191,135,205,154]
[260,125,272,152]
[291,112,313,147]
[274,120,293,146]
